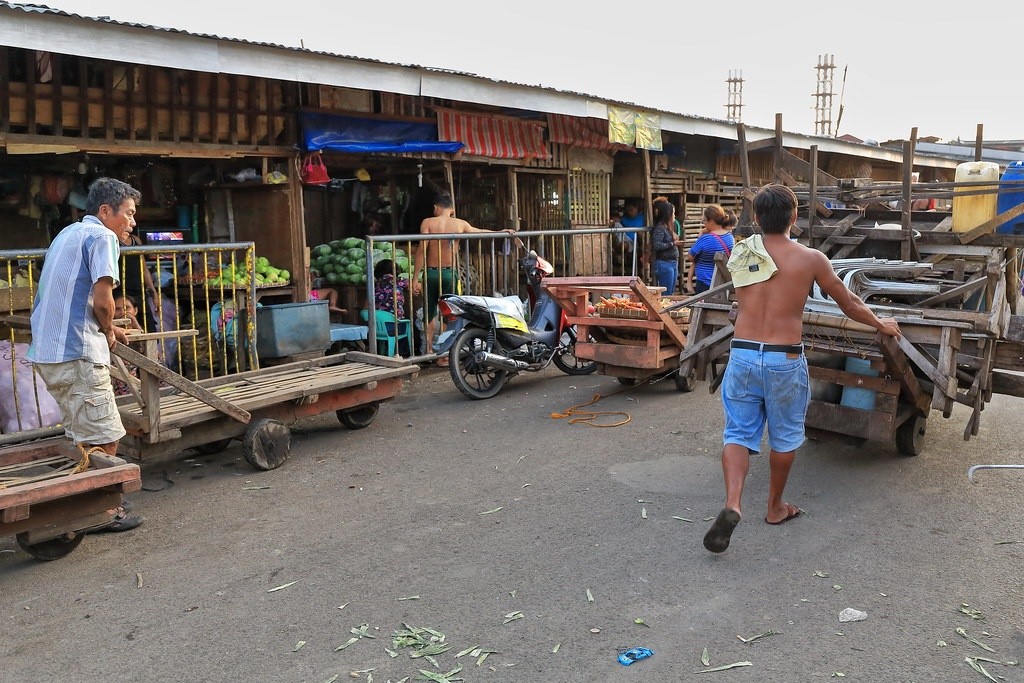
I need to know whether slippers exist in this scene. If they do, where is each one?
[765,506,801,526]
[703,509,741,554]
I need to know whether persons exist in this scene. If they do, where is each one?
[113,234,160,362]
[686,205,738,295]
[309,289,347,314]
[912,198,935,210]
[609,197,684,296]
[111,294,145,355]
[25,177,145,534]
[703,184,901,553]
[363,258,422,357]
[411,196,516,367]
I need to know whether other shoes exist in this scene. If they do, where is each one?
[86,513,145,535]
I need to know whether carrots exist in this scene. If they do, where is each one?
[599,296,647,310]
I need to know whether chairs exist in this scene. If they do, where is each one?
[360,309,413,359]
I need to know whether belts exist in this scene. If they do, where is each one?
[731,340,803,353]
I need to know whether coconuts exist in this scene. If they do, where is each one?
[209,257,290,284]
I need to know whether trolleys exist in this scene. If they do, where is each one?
[539,275,706,393]
[0,424,143,562]
[108,327,422,472]
[726,299,933,457]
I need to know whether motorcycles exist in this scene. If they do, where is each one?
[431,236,602,401]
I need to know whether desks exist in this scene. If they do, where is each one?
[328,320,369,352]
[168,284,293,372]
